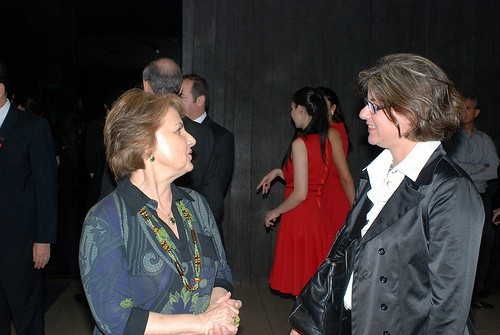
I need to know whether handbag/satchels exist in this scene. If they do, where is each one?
[286,222,355,335]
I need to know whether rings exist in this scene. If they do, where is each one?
[232,316,239,326]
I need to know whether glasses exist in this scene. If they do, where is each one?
[363,96,391,114]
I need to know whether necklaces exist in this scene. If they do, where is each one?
[158,192,175,225]
[141,199,201,290]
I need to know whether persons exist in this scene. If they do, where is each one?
[0,59,243,335]
[255,54,500,335]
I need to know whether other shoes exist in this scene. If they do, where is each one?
[473,301,494,308]
